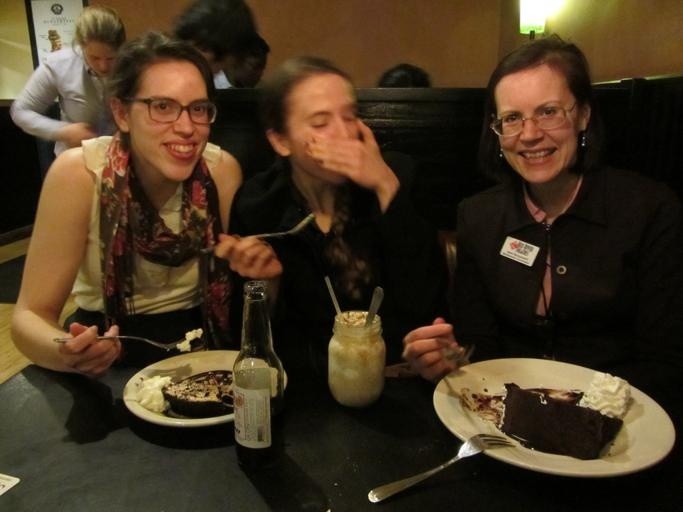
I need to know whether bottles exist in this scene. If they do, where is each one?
[232,280,284,457]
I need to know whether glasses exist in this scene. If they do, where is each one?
[489,102,577,136]
[126,98,217,124]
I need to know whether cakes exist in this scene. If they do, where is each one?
[175,327,204,353]
[500,369,632,460]
[136,364,234,415]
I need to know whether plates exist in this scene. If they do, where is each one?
[123,350,268,427]
[433,357,676,478]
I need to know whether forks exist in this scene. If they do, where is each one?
[435,338,475,369]
[368,433,516,503]
[52,328,185,352]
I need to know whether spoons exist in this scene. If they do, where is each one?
[200,214,315,254]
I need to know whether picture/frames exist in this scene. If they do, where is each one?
[24,0,89,72]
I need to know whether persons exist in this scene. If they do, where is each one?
[6,5,132,160]
[10,29,242,379]
[402,37,681,386]
[169,2,272,89]
[379,61,437,84]
[213,54,449,376]
